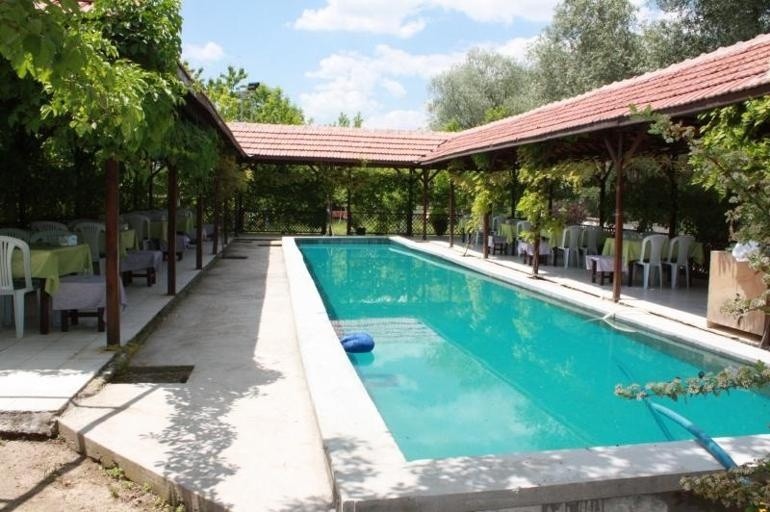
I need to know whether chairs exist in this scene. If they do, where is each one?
[0,209,193,342]
[494,216,696,292]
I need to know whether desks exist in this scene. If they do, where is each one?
[194,226,207,242]
[488,234,506,256]
[706,248,770,336]
[206,223,216,236]
